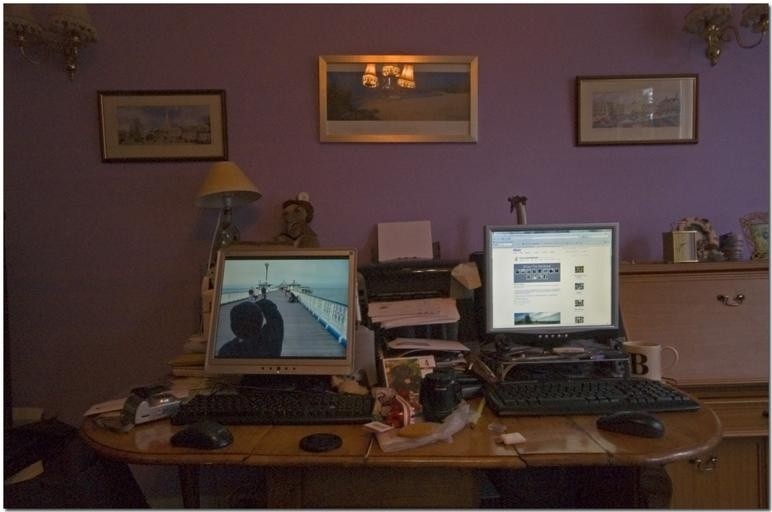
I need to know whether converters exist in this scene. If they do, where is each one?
[496,433,525,450]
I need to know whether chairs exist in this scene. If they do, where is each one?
[4,409,151,509]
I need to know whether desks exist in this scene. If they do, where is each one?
[82,377,721,509]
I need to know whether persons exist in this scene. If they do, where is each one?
[219,284,298,357]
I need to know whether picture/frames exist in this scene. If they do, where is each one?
[673,216,717,262]
[577,73,699,147]
[317,53,478,144]
[95,89,229,163]
[738,212,770,261]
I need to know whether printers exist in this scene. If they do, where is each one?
[358,241,475,312]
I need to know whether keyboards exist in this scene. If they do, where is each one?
[486,377,702,416]
[170,393,375,423]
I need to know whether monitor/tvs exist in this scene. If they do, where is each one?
[484,222,620,361]
[203,246,358,391]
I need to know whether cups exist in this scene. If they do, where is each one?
[623,341,680,379]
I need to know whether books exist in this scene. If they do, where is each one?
[377,219,434,262]
[169,337,210,396]
[83,397,135,432]
[368,296,460,328]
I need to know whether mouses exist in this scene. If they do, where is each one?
[169,423,234,449]
[596,410,665,438]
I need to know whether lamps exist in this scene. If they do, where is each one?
[682,4,770,68]
[192,161,262,247]
[4,12,96,81]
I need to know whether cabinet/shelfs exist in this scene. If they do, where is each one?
[264,468,473,509]
[615,262,770,386]
[664,384,768,508]
